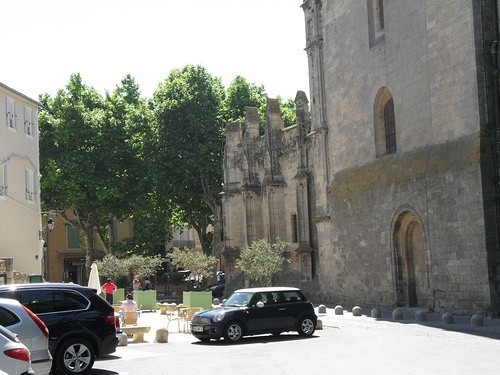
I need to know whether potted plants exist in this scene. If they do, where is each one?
[96,253,164,311]
[165,246,220,312]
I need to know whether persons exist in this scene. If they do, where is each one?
[101,278,117,305]
[118,293,139,327]
[131,279,151,291]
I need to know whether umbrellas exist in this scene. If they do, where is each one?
[87,261,102,296]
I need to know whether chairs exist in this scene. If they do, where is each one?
[252,293,262,306]
[266,293,275,304]
[119,311,141,338]
[162,307,204,334]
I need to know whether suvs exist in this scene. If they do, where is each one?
[0,282,120,375]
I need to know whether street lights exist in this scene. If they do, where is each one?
[46,218,55,281]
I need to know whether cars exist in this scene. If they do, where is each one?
[0,324,36,375]
[189,286,318,345]
[0,297,53,375]
